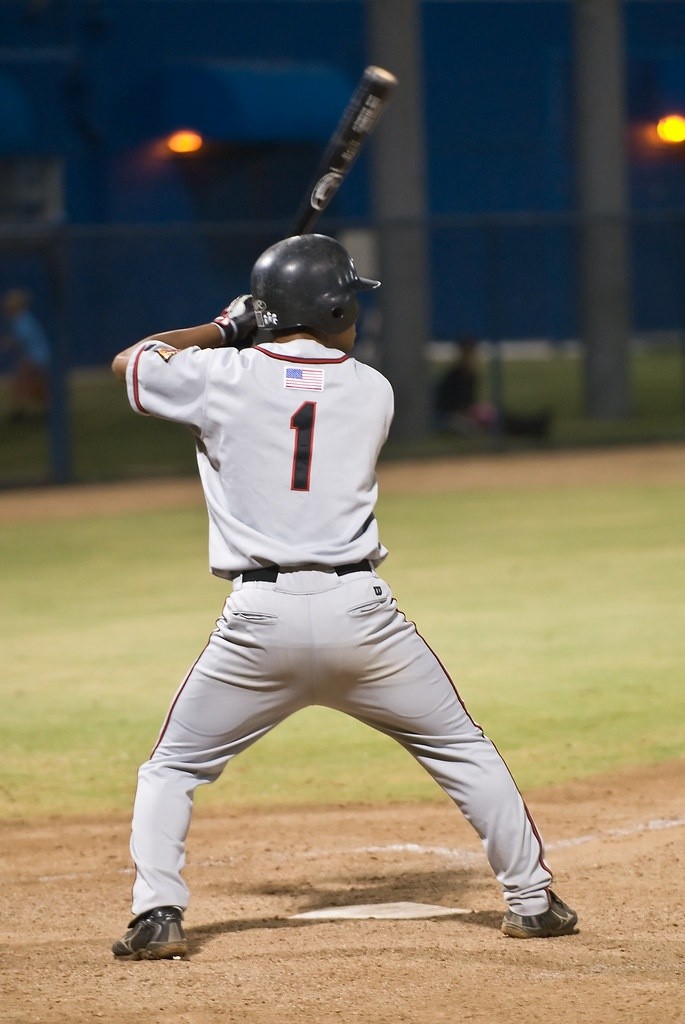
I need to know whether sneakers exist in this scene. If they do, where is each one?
[112,908,189,959]
[500,889,580,939]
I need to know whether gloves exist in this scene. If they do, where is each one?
[211,294,261,346]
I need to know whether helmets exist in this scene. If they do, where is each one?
[252,235,381,334]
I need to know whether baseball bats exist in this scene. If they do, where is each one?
[246,63,399,349]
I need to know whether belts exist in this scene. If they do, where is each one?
[242,560,372,583]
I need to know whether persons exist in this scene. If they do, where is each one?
[111,231,580,961]
[0,272,75,482]
[430,338,502,449]
